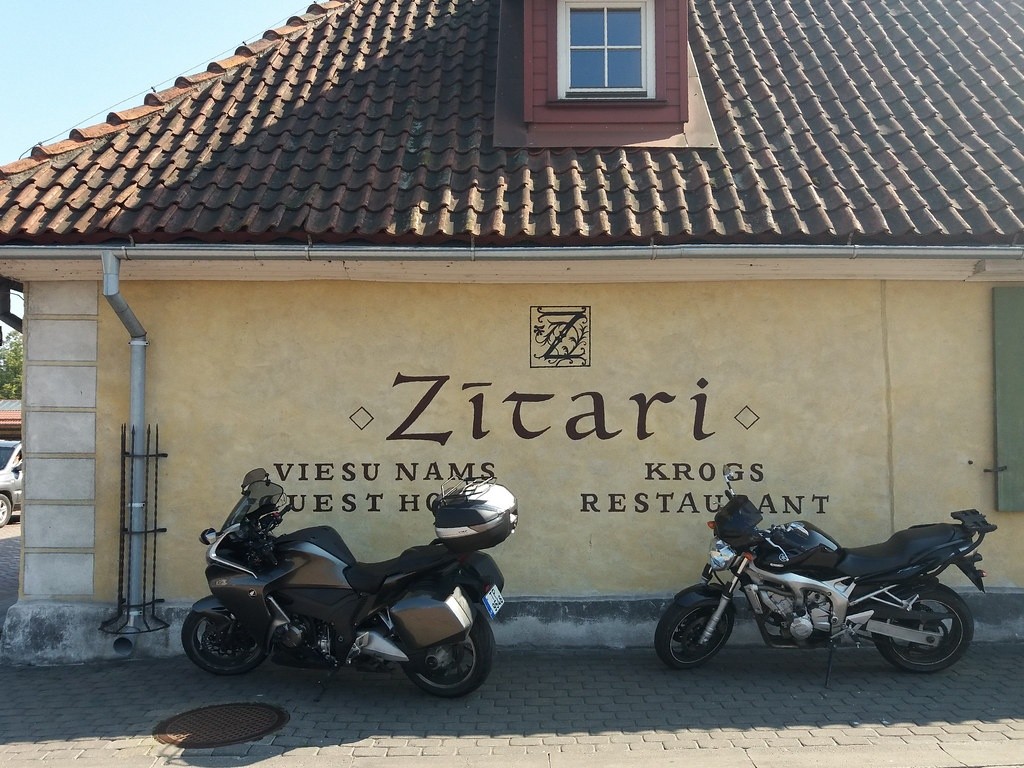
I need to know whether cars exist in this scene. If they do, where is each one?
[0,439,24,528]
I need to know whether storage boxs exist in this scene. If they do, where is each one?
[437,484,519,551]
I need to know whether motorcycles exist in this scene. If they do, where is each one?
[655,463,1000,686]
[178,467,519,699]
